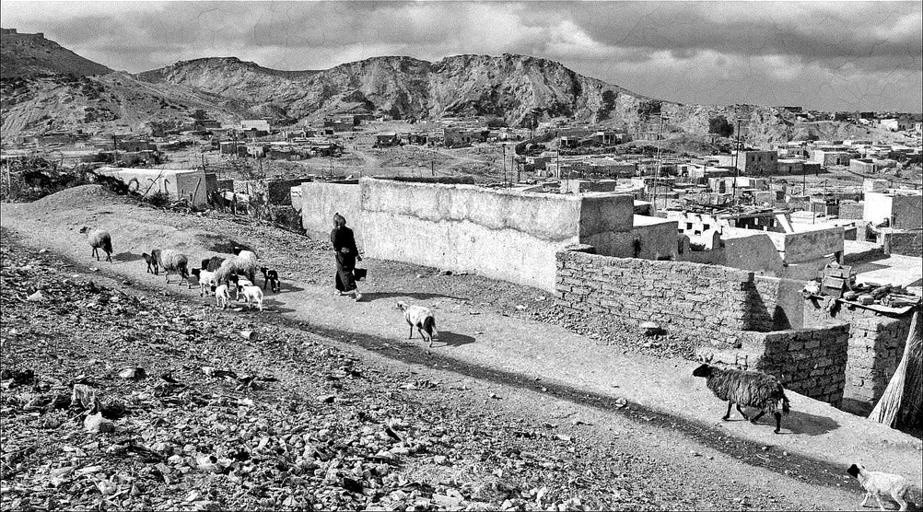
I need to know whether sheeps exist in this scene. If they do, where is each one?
[846,460,913,512]
[79,225,119,263]
[151,248,195,289]
[192,241,282,312]
[141,251,160,276]
[691,360,791,434]
[393,300,439,347]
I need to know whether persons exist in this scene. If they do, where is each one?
[331,214,364,301]
